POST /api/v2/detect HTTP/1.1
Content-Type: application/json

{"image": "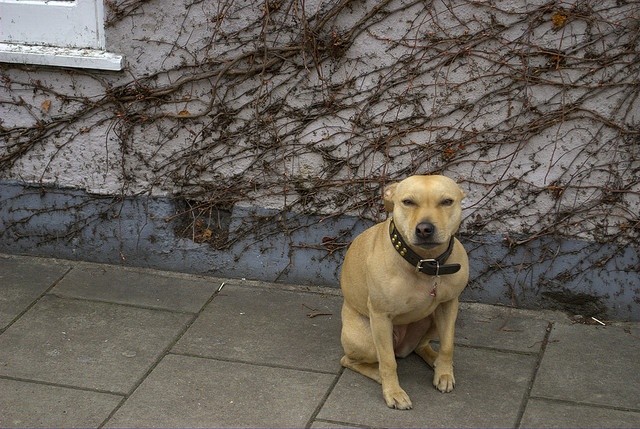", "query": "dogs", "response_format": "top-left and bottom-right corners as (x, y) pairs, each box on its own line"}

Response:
(338, 173), (470, 410)
